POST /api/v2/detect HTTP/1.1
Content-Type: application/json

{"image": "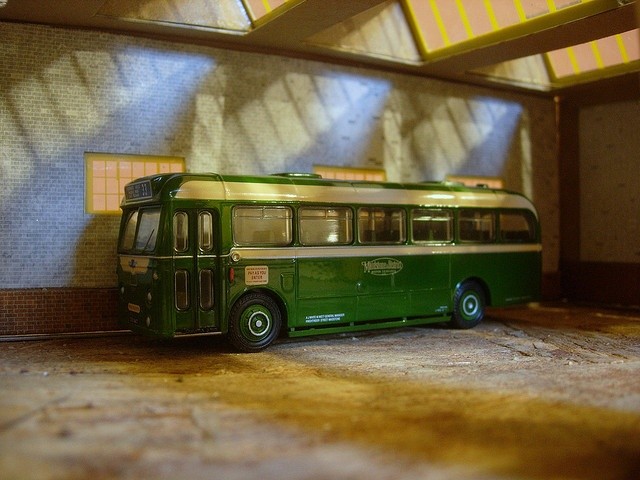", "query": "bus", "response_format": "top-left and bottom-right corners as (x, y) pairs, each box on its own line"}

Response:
(116, 171), (544, 353)
